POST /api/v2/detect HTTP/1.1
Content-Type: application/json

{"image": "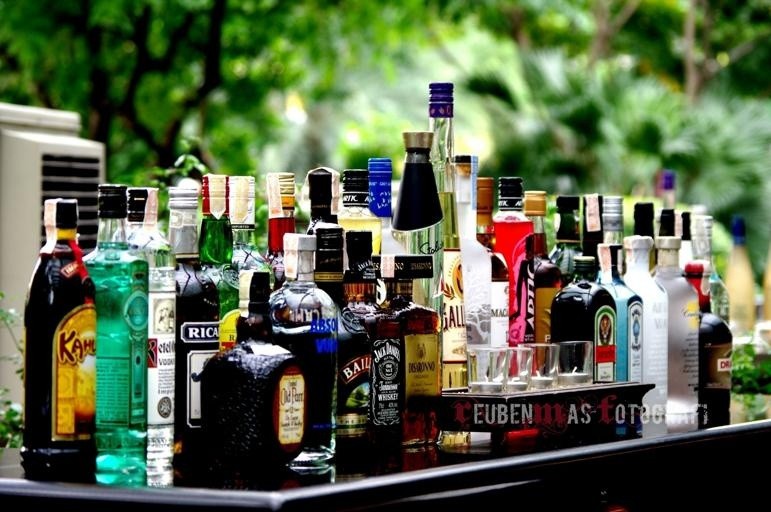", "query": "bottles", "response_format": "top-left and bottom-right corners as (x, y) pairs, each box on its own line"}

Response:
(19, 82), (755, 490)
